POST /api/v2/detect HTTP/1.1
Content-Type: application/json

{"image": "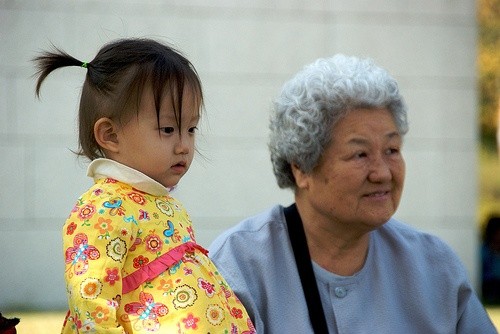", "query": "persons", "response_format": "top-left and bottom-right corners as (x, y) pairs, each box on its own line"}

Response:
(211, 53), (498, 334)
(30, 37), (258, 334)
